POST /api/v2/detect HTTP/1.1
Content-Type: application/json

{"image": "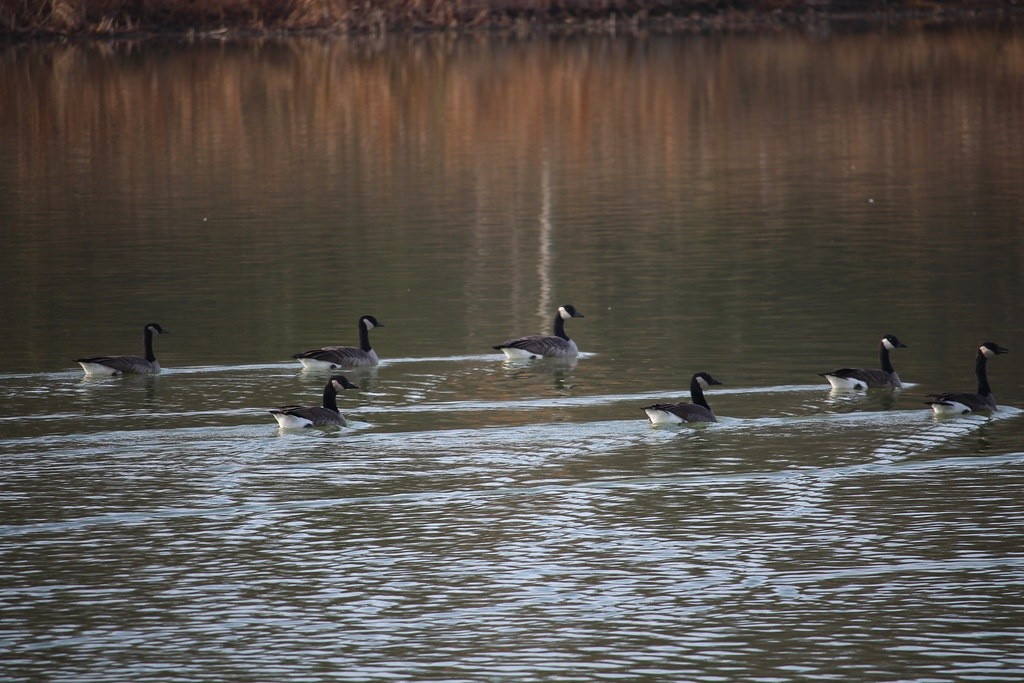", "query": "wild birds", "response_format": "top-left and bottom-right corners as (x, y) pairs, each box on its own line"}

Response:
(640, 371), (723, 423)
(71, 321), (170, 378)
(288, 314), (386, 370)
(492, 303), (585, 362)
(818, 334), (909, 392)
(268, 374), (360, 431)
(922, 341), (1012, 428)
